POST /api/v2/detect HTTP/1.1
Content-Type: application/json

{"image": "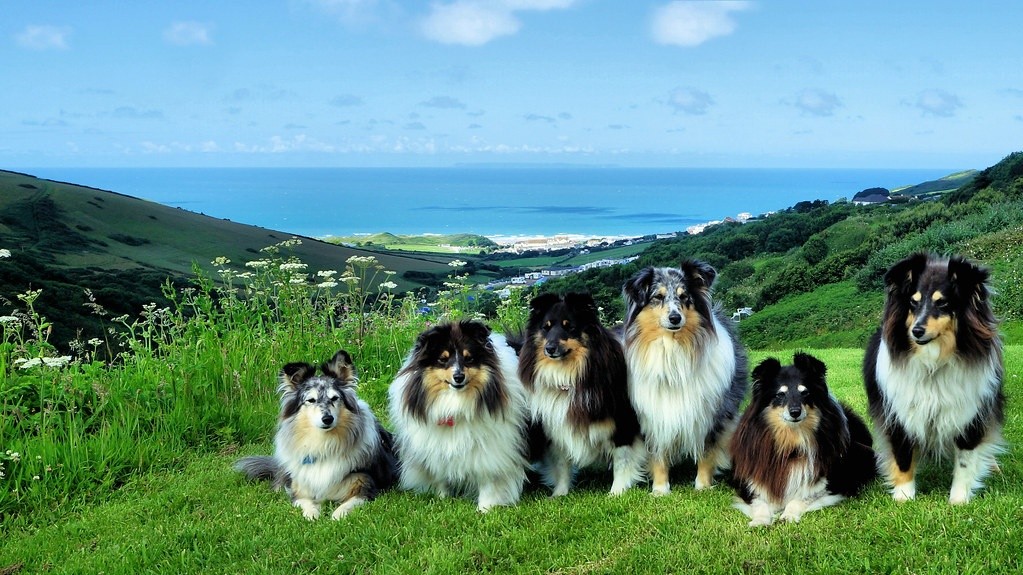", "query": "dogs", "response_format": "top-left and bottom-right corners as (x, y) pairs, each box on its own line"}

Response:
(518, 289), (651, 497)
(231, 348), (402, 522)
(862, 248), (1013, 507)
(619, 258), (749, 498)
(721, 350), (877, 526)
(387, 318), (536, 513)
